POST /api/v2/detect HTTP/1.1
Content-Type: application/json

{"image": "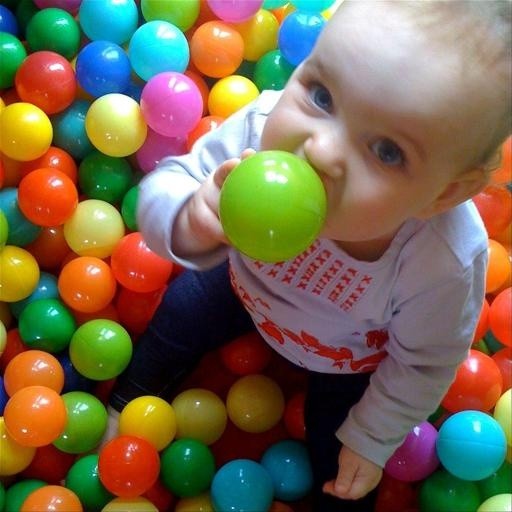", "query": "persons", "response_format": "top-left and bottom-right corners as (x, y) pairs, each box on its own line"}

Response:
(86, 2), (512, 511)
